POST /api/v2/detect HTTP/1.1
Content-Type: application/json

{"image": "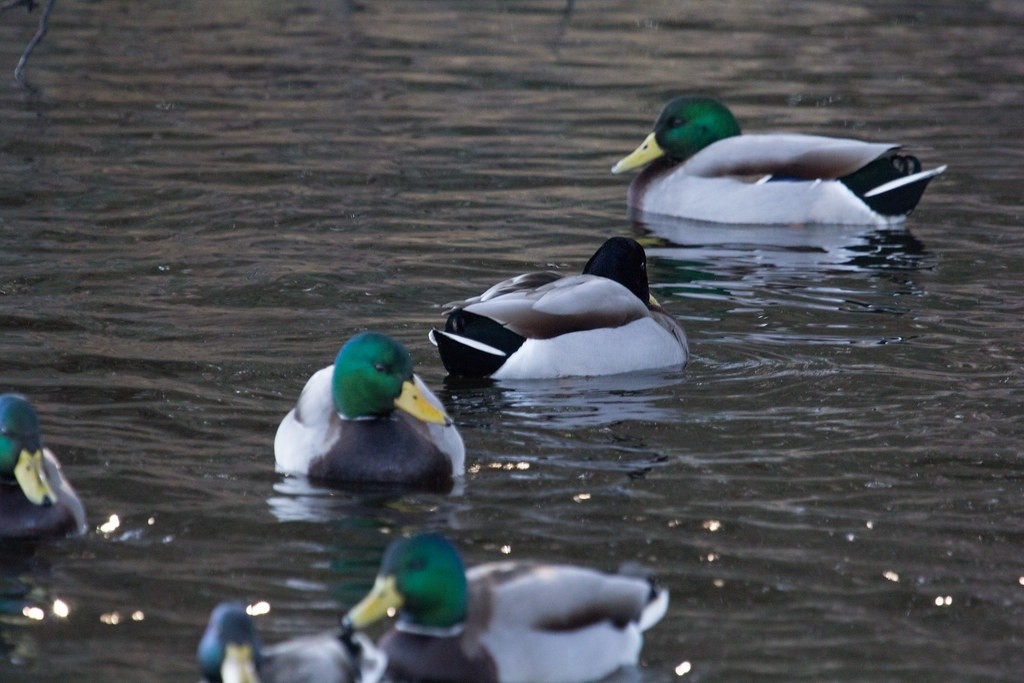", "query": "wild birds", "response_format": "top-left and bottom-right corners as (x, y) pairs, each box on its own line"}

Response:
(0, 393), (86, 539)
(610, 96), (948, 225)
(342, 532), (670, 683)
(427, 237), (689, 379)
(196, 600), (389, 683)
(271, 333), (466, 486)
